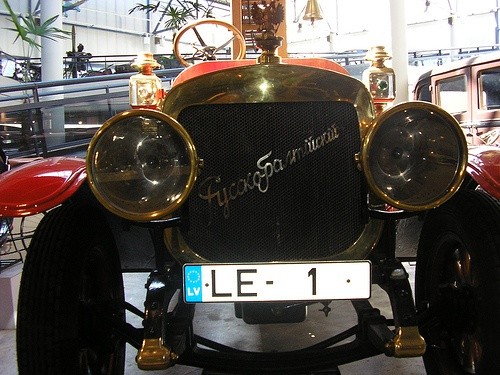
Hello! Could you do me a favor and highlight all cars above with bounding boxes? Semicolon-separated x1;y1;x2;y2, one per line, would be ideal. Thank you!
0;0;500;373
419;54;500;204
